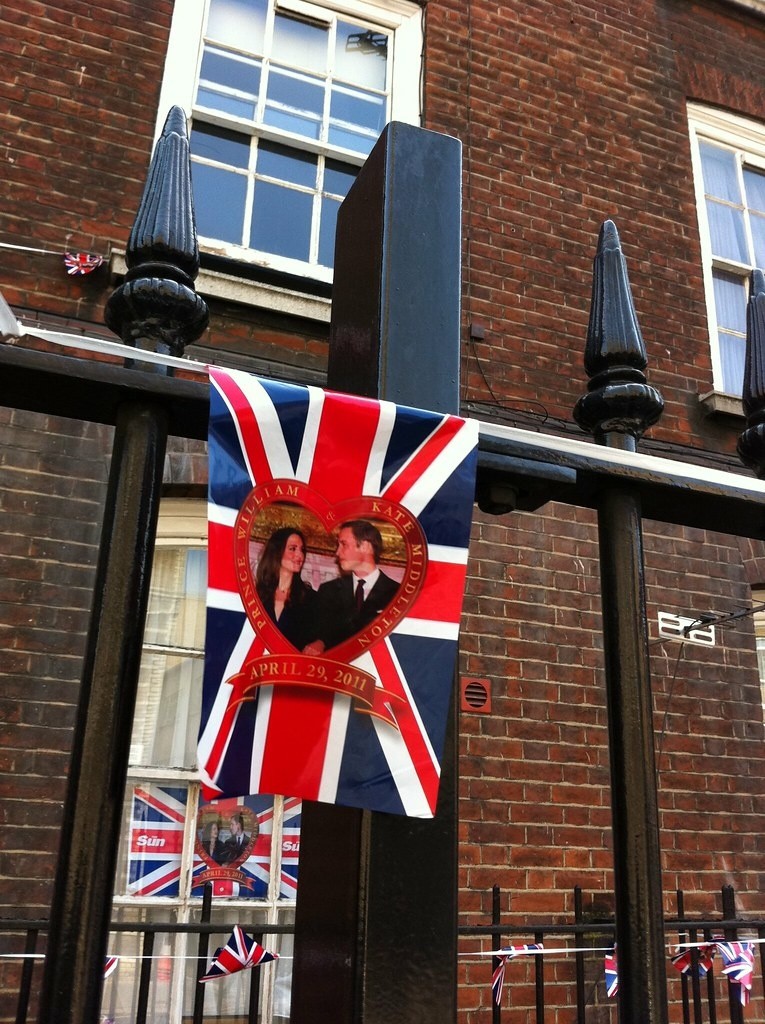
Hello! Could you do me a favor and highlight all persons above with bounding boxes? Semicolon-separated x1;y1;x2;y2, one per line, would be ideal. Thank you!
202;815;251;868
254;520;403;658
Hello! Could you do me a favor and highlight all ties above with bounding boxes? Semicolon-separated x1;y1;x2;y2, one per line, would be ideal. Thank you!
237;836;241;847
355;578;366;616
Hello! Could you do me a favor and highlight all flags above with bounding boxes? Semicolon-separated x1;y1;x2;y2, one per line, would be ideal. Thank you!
717;943;754;1005
491;943;543;1005
195;366;478;819
125;782;302;898
671;936;725;978
605;951;618;998
199;923;280;984
64;252;103;276
